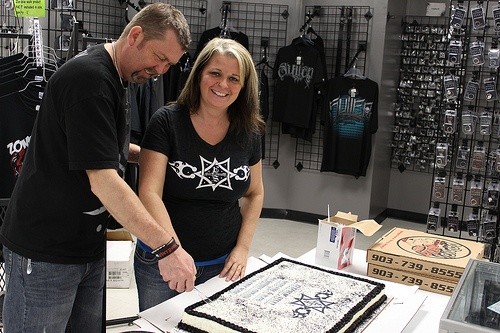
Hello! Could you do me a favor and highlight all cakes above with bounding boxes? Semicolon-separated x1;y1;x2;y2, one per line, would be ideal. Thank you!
176;257;387;333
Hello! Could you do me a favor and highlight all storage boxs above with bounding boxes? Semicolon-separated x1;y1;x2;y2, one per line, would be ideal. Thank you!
367;227;484;297
439;258;500;333
316;212;383;270
105;231;135;290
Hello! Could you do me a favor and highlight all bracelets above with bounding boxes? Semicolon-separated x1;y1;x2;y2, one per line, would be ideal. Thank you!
151;236;180;260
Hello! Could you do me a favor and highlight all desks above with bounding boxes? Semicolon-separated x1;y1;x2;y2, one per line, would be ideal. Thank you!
297;247;452;333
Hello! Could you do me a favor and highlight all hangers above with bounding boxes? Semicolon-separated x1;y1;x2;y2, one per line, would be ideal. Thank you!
344;57;365;79
254;56;273;71
20;23;61;92
219;20;238;39
292;18;317;45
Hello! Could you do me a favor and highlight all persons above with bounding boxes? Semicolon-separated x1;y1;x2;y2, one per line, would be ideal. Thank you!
132;37;265;312
0;1;197;331
